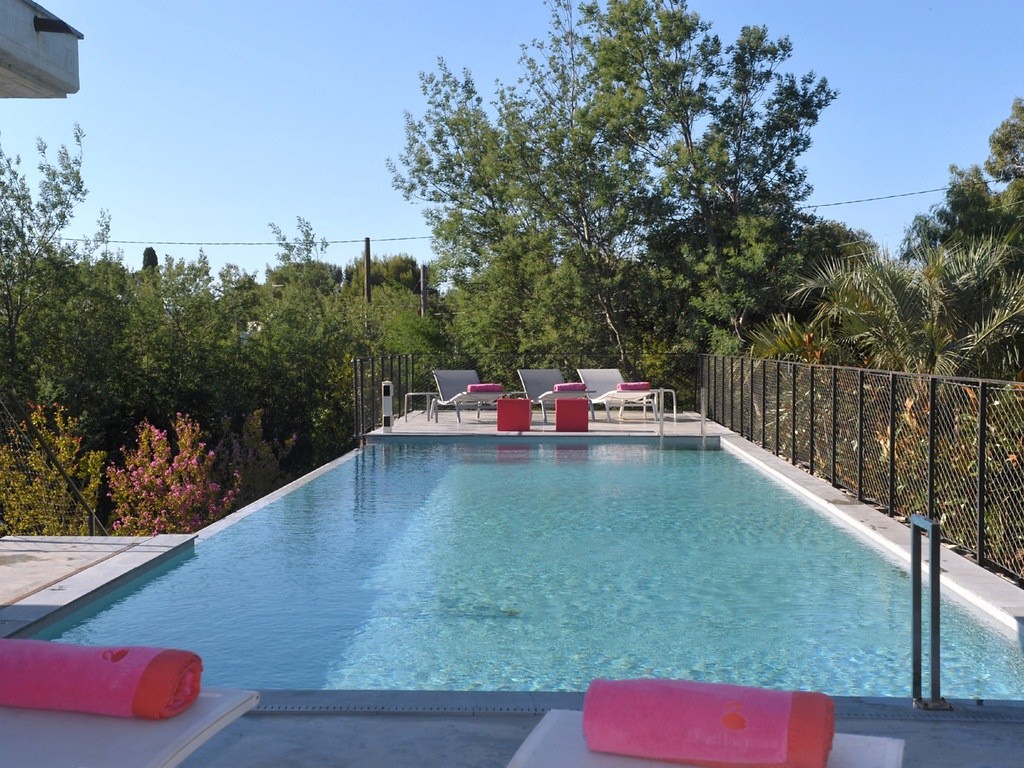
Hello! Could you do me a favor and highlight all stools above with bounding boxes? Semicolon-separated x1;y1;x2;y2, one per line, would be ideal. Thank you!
555;398;588;432
496;398;530;431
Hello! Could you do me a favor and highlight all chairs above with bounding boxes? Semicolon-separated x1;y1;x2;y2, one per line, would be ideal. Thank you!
424;368;530;424
0;685;261;768
499;706;907;768
517;369;597;425
575;369;678;423
